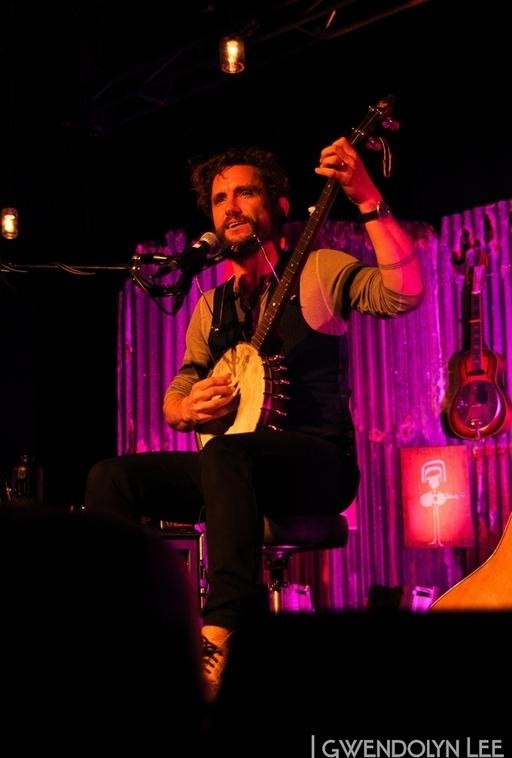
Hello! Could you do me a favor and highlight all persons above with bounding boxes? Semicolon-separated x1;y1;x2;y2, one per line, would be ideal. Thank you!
90;136;428;700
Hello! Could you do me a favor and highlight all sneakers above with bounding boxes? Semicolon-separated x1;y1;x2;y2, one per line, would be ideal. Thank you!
201;632;230;702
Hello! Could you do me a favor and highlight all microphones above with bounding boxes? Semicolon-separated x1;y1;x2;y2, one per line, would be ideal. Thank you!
150;228;219;280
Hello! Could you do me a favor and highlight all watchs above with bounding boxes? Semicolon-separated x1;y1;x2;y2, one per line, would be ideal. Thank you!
350;197;391;223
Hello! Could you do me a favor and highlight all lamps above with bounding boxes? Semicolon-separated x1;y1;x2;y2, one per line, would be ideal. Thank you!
218;35;247;76
1;207;19;242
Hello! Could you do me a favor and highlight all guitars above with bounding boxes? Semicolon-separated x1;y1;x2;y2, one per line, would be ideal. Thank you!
447;249;511;441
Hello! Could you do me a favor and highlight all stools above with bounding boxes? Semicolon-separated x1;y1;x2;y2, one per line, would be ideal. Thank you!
260;512;352;616
